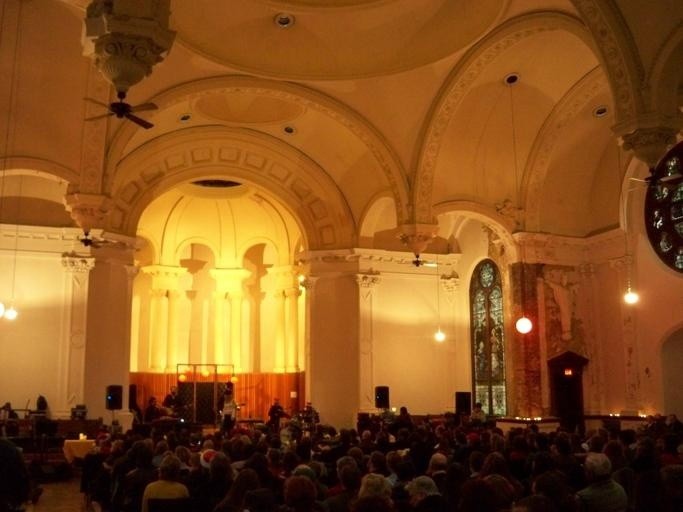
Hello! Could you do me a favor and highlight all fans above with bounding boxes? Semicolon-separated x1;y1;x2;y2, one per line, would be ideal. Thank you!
77;0;175;132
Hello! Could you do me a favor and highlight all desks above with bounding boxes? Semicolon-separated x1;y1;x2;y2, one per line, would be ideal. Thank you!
64;439;96;468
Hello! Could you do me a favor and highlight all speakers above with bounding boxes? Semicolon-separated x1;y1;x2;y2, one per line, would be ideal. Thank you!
106;385;122;409
375;386;389;408
